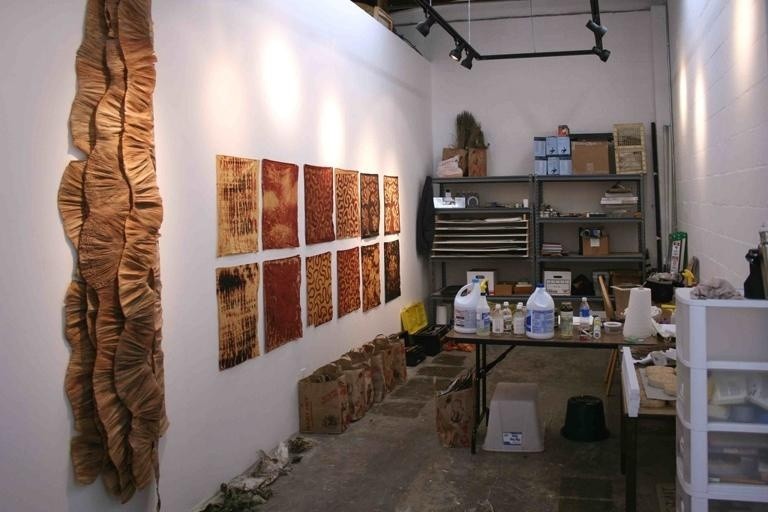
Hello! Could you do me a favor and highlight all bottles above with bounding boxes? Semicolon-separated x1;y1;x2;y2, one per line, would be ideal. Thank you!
477;292;490;336
560;302;573;338
503;302;512;331
492;304;504;336
594;317;601;339
513;305;525;335
555;308;558;327
518;302;526;314
579;297;589;329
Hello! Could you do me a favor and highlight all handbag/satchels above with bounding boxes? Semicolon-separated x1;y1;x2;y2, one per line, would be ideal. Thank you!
297;363;350;434
373;333;407;384
312;357;365;422
372;342;395;393
442;148;466;175
468;147;486;178
350;342;385;403
437;379;476;449
335;348;374;411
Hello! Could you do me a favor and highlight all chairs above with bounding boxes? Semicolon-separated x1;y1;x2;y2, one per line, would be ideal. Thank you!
597;275;624;396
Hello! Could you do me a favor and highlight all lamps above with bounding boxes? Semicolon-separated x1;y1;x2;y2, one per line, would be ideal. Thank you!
415;15;473;70
586;17;611;63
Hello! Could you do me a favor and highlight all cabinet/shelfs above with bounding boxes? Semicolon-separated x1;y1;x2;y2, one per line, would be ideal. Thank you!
674;287;768;512
428;173;534;324
535;176;646;311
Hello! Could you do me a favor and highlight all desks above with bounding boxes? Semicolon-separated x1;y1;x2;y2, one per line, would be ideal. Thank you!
445;317;676;453
619;365;677;512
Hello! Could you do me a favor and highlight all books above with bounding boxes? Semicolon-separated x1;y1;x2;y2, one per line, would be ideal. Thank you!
708;436;768;481
599;191;638;210
542;242;563;254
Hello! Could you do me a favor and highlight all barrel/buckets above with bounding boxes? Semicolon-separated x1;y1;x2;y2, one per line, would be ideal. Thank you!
453;279;481;333
525;284;555;340
560;396;610;442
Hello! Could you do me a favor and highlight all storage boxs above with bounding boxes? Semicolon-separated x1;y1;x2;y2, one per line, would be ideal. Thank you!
402;303;429;334
443;146;487;176
434;377;478;446
542;269;572;297
600;204;640;218
415;326;448;355
481;382;546;453
494;280;514;296
467;268;498;295
570;141;610;176
592;268;644;315
581;235;609;256
514;281;533;294
